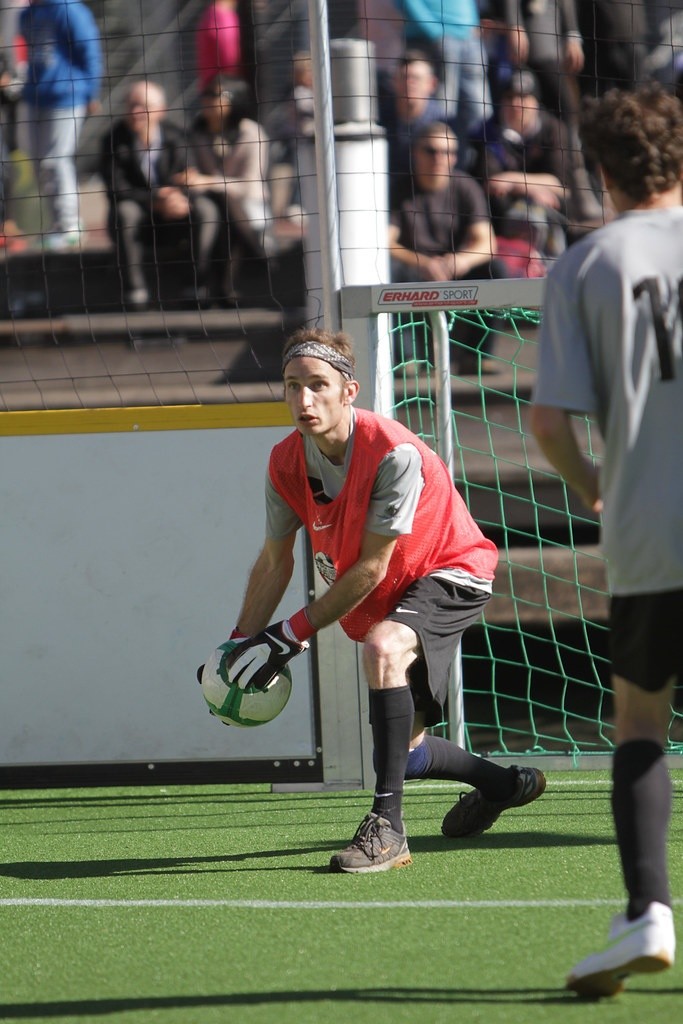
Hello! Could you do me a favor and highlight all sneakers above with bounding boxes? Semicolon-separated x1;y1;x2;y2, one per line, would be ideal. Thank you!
442;765;545;840
329;808;414;873
566;901;675;997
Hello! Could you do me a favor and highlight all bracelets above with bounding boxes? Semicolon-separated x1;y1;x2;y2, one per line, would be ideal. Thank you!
230;629;248;639
289;608;319;643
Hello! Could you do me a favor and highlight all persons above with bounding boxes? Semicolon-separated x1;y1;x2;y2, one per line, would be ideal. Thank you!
0;0;683;377
197;327;546;874
531;85;683;993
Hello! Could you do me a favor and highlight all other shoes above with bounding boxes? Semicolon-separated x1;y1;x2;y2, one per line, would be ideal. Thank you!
396;358;420;378
457;359;498;374
34;226;84;253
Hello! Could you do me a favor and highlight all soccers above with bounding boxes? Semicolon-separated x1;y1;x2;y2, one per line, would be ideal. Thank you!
200;636;292;727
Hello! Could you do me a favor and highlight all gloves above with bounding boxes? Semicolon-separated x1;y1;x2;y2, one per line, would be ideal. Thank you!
197;628;248;686
226;607;319;692
